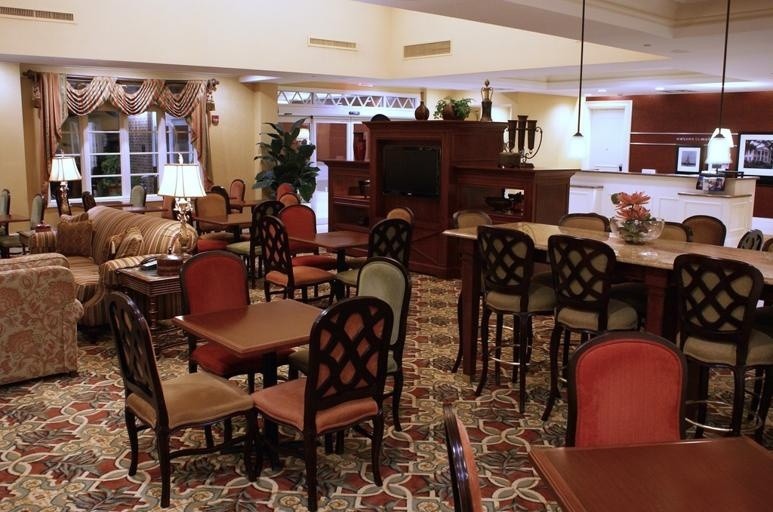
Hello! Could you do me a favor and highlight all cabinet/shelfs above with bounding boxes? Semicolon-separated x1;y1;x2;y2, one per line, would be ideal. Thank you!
319;158;370;257
449;161;581;277
355;116;509;278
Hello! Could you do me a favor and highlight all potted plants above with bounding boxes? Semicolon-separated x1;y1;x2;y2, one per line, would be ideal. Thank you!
434;93;476;118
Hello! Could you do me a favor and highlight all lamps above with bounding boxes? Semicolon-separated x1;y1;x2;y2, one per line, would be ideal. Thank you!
564;0;591;159
47;149;82;215
703;0;732;167
157;154;207;260
711;0;735;148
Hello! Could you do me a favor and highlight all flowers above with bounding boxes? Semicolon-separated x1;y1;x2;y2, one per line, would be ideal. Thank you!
615;193;651;223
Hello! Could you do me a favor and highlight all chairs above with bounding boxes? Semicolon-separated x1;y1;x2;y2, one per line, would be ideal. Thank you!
0;190;46;257
104;292;256;508
443;407;484;512
566;330;687;447
735;229;772;252
259;205;413;302
476;226;559;414
189;178;305;291
675;253;773;440
541;236;638;422
452;210;505;374
249;296;396;512
660;221;692;240
82;185;168;213
180;250;294;452
287;257;412;433
555;213;610;231
683;215;729;246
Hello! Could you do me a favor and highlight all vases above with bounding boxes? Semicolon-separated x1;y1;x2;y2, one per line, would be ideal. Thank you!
611;219;664;244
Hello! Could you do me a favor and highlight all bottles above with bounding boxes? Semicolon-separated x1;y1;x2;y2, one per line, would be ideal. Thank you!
352;131;368;160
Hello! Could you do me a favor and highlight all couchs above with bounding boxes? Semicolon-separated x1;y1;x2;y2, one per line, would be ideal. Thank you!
0;254;85;387
27;205;198;343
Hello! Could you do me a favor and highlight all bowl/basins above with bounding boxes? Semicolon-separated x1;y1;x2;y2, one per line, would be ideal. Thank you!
483;197;512;211
609;216;664;245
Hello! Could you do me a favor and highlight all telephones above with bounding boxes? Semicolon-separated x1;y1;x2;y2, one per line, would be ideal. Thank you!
140;256;157;271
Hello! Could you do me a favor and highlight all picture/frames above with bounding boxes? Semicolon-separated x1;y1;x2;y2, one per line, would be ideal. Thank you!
674;144;703;174
736;130;772;187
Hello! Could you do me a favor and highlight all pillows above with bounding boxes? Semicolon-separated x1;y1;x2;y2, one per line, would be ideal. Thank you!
99;234;122;261
115;224;145;259
54;221;94;258
60;212;88;222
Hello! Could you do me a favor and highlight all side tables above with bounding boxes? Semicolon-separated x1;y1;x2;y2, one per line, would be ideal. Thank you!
114;263;183;340
